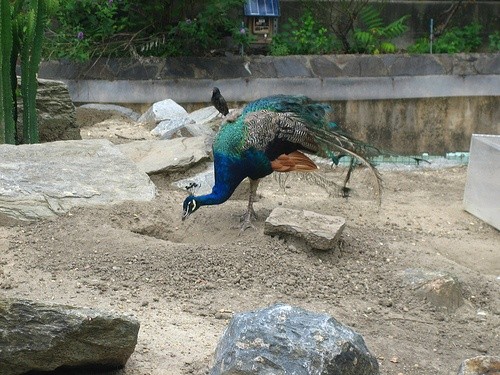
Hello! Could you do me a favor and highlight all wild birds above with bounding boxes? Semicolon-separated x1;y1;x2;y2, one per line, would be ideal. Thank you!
207;86;230;118
183;94;432;236
